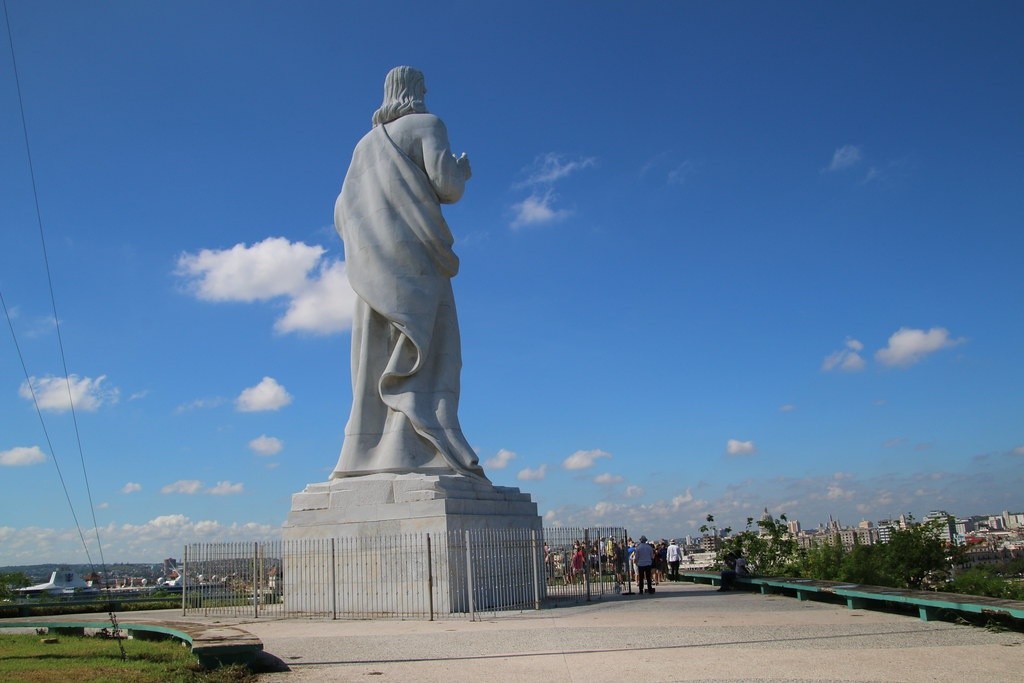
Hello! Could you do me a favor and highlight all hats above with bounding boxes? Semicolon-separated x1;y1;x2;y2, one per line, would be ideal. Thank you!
639;535;648;541
669;538;675;543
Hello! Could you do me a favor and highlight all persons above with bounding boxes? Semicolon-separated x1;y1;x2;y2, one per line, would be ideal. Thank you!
543;533;748;595
326;64;494;489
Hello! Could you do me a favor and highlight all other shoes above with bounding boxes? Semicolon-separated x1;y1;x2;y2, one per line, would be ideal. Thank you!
648;590;654;593
638;590;643;594
